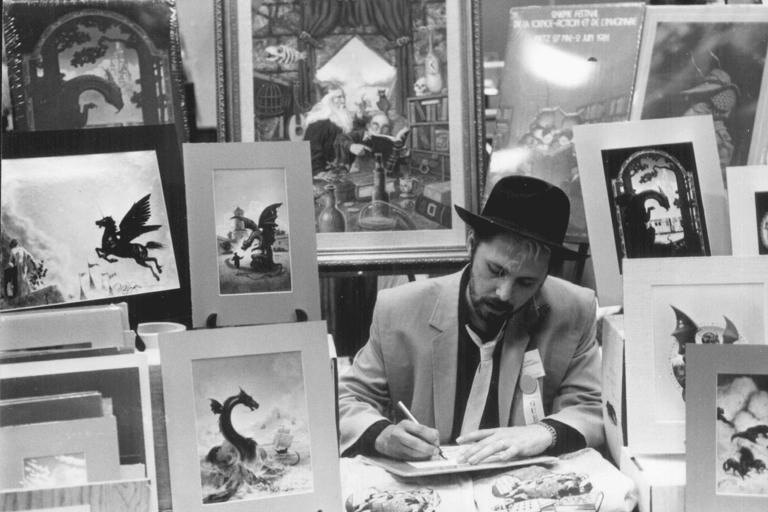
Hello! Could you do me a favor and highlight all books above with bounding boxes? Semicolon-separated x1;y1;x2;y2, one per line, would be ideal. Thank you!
0;303;160;512
366;125;410;175
404;98;448;153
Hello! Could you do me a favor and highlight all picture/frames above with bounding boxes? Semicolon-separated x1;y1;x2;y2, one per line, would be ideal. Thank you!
205;0;487;266
1;148;183;313
570;113;730;309
620;255;768;457
178;140;327;330
683;342;768;512
157;320;345;510
724;162;768;255
4;1;193;148
630;0;768;166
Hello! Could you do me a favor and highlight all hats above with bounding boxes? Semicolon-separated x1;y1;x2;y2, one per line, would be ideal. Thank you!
454;175;589;260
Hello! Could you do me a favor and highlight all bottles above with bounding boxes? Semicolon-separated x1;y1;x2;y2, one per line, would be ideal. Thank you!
423;25;445;94
369;151;390;218
317;183;347;233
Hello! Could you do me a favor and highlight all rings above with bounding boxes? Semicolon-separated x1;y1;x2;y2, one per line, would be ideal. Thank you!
498;439;506;451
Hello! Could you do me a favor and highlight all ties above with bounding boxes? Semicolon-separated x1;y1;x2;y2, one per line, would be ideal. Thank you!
460;320;510;443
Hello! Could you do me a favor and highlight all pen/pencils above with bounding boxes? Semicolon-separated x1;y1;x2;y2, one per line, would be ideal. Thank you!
396;401;445;459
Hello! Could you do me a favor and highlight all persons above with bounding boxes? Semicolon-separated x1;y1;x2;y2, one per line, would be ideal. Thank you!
301;86;364;177
336;174;602;468
331;113;395;176
1;237;41;300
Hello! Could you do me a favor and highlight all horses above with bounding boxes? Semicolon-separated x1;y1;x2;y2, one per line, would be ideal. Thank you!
96;217;168;280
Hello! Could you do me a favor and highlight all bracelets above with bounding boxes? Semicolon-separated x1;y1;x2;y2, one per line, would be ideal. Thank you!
530;419;557;452
